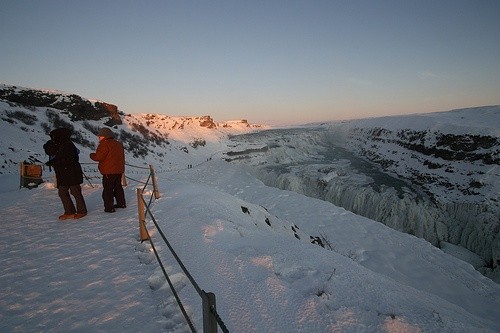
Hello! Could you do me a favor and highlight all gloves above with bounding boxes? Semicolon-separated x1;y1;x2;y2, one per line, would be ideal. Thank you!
45;160;54;166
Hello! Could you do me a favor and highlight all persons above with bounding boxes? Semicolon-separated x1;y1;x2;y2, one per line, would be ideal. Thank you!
42;127;90;222
88;127;127;214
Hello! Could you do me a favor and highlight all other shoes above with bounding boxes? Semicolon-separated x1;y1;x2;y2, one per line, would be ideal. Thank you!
59;213;76;220
104;209;115;212
114;205;127;208
74;213;87;219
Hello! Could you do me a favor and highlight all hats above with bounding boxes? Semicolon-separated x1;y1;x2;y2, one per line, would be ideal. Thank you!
97;128;114;136
